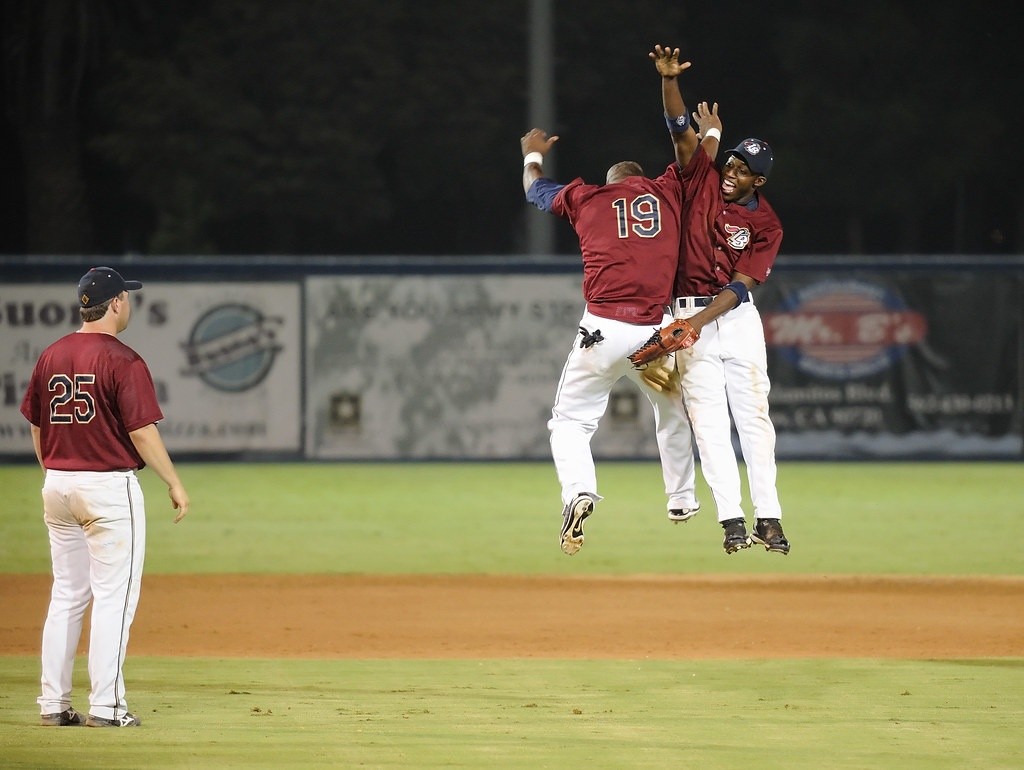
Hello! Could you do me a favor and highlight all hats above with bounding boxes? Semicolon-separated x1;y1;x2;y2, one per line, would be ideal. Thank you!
724;138;775;178
77;266;143;308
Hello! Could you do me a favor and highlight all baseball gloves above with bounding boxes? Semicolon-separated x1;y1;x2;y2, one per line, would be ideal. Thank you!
627;318;702;369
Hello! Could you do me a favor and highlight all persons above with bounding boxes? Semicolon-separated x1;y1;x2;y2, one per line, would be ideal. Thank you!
520;103;721;556
26;266;190;730
647;43;791;554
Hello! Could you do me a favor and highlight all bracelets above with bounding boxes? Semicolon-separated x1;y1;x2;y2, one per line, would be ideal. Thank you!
705;128;721;142
524;152;543;168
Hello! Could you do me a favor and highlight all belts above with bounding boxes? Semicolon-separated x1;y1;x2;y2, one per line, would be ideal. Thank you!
677;293;752;307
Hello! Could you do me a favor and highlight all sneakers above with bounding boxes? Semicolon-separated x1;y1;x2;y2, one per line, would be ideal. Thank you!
40;706;87;726
560;493;595;557
722;517;750;554
667;499;701;524
749;518;790;555
86;711;141;727
